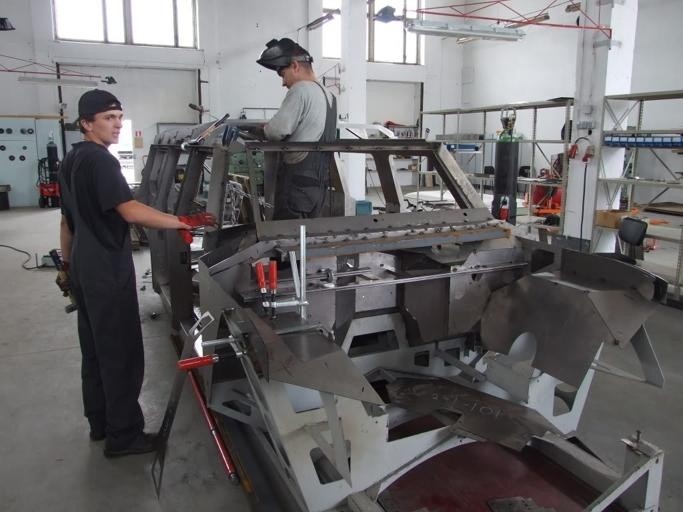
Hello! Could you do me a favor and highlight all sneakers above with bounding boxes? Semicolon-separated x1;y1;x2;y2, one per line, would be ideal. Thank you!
102;431;161;459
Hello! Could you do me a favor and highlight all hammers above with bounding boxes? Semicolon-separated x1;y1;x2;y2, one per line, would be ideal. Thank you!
49;249;79;313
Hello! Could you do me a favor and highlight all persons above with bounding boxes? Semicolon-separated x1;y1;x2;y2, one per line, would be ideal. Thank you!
257;44;345;219
54;90;214;456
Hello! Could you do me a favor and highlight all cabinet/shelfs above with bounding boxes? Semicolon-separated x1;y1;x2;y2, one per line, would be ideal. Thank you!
419;89;683;304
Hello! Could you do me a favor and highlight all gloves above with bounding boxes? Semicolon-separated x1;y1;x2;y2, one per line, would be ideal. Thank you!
177;211;217;229
55;261;73;297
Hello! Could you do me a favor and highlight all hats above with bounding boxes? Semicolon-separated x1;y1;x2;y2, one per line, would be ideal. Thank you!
76;88;123;123
